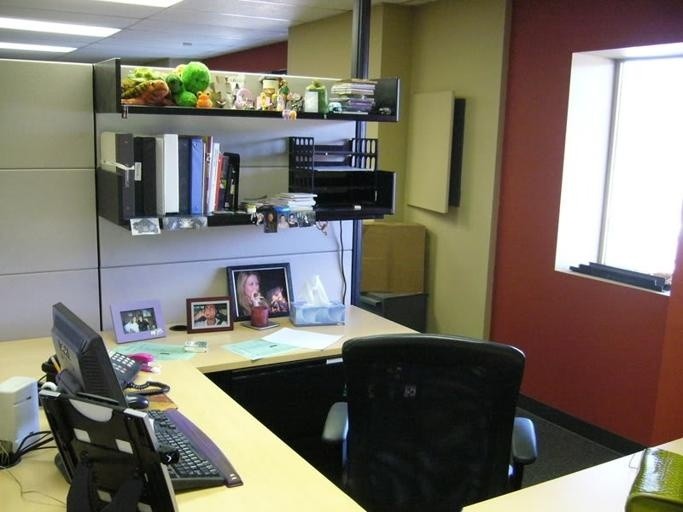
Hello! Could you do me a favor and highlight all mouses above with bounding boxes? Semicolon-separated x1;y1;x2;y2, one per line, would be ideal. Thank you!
123;393;149;409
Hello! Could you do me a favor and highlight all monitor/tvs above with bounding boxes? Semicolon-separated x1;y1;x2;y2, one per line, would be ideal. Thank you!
39;390;180;512
50;302;128;485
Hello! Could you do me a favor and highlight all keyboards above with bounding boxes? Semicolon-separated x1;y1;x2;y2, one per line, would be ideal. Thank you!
140;408;242;490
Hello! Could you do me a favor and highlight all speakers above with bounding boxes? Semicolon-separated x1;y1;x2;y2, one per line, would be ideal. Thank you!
0;376;40;452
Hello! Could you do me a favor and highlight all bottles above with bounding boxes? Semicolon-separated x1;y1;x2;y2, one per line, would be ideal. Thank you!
305;81;329;113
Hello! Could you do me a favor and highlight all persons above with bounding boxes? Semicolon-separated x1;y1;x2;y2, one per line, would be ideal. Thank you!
124;316;139;333
138;314;149;331
265;213;276;232
194;304;227;327
237;271;271;316
302;213;311;226
289;213;298;227
278;215;289;228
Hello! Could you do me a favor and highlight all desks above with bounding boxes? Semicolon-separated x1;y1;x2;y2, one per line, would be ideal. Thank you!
0;302;683;512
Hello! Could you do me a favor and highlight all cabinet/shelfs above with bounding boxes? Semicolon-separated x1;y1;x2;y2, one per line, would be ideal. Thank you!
92;56;399;232
355;292;428;331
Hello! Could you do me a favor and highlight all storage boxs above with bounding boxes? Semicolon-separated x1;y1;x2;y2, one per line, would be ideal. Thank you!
362;218;428;295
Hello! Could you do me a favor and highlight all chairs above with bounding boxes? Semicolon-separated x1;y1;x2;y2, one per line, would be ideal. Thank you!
321;333;538;512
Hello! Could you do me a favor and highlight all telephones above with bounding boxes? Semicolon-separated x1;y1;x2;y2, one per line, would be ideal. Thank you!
42;346;140;392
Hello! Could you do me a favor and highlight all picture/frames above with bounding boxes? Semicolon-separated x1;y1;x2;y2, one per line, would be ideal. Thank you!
109;298;166;344
226;262;294;322
185;297;234;332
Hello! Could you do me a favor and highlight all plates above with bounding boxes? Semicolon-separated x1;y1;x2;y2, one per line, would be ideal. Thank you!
242;319;279;330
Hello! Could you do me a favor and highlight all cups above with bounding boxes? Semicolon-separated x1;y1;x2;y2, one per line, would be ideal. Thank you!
262;80;279;103
302;91;319;113
250;306;269;326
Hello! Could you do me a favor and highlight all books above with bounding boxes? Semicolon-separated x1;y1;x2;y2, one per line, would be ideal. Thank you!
272;192;318;211
328;78;379;115
100;130;236;220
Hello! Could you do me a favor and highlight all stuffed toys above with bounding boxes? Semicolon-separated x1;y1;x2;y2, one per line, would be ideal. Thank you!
121;61;213;108
